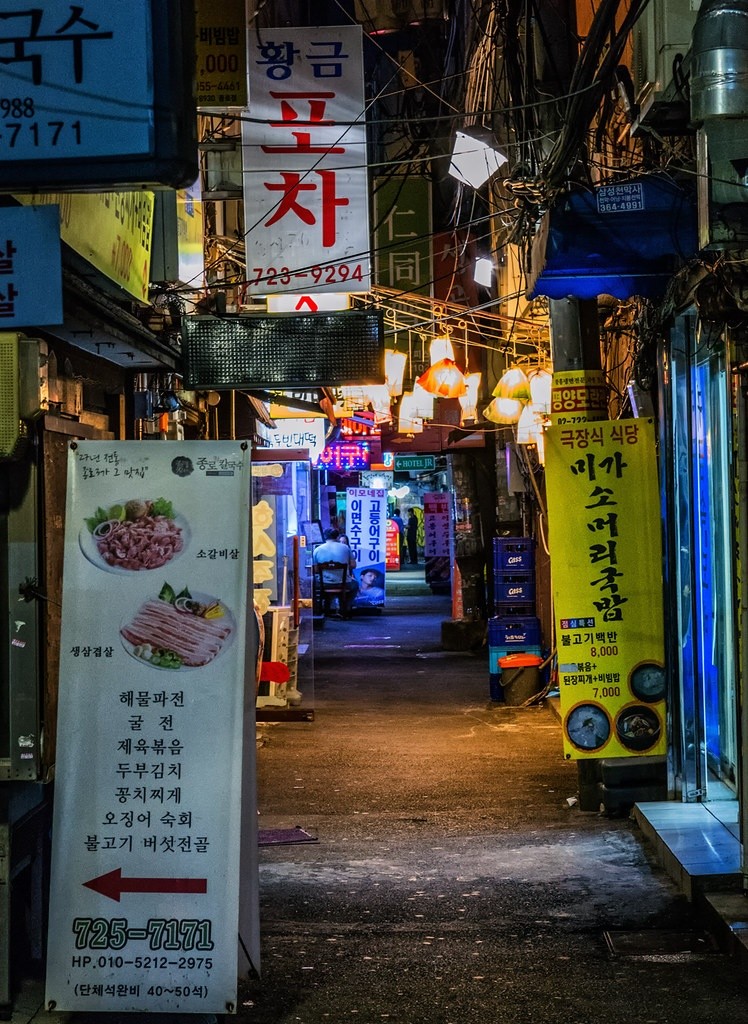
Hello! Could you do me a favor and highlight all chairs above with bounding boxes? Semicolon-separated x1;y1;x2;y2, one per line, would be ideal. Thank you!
313;559;348;617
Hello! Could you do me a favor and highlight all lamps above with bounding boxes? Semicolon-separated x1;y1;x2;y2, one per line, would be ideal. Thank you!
472;250;496;288
447;124;509;190
346;309;549;465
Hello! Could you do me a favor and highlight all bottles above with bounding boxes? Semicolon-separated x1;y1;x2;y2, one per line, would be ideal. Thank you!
493;608;531;629
505;544;527;552
504;576;528;583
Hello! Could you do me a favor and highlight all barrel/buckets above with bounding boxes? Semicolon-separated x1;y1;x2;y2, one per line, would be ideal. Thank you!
499;667;539;705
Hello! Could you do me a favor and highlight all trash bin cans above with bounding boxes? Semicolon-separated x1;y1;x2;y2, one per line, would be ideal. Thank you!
498;653;544;706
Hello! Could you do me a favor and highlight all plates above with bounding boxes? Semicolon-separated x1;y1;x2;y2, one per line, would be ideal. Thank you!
120;591;236;672
79;499;192;575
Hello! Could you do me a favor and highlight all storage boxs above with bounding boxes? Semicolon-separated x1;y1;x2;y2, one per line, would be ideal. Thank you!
486;535;541;698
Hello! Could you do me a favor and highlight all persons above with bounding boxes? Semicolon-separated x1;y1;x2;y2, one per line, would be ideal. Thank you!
355;568;384;605
312;527;360;622
405;508;418;564
390;509;405;565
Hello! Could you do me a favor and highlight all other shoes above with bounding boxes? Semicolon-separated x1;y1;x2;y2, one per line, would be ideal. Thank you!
407;561;417;564
